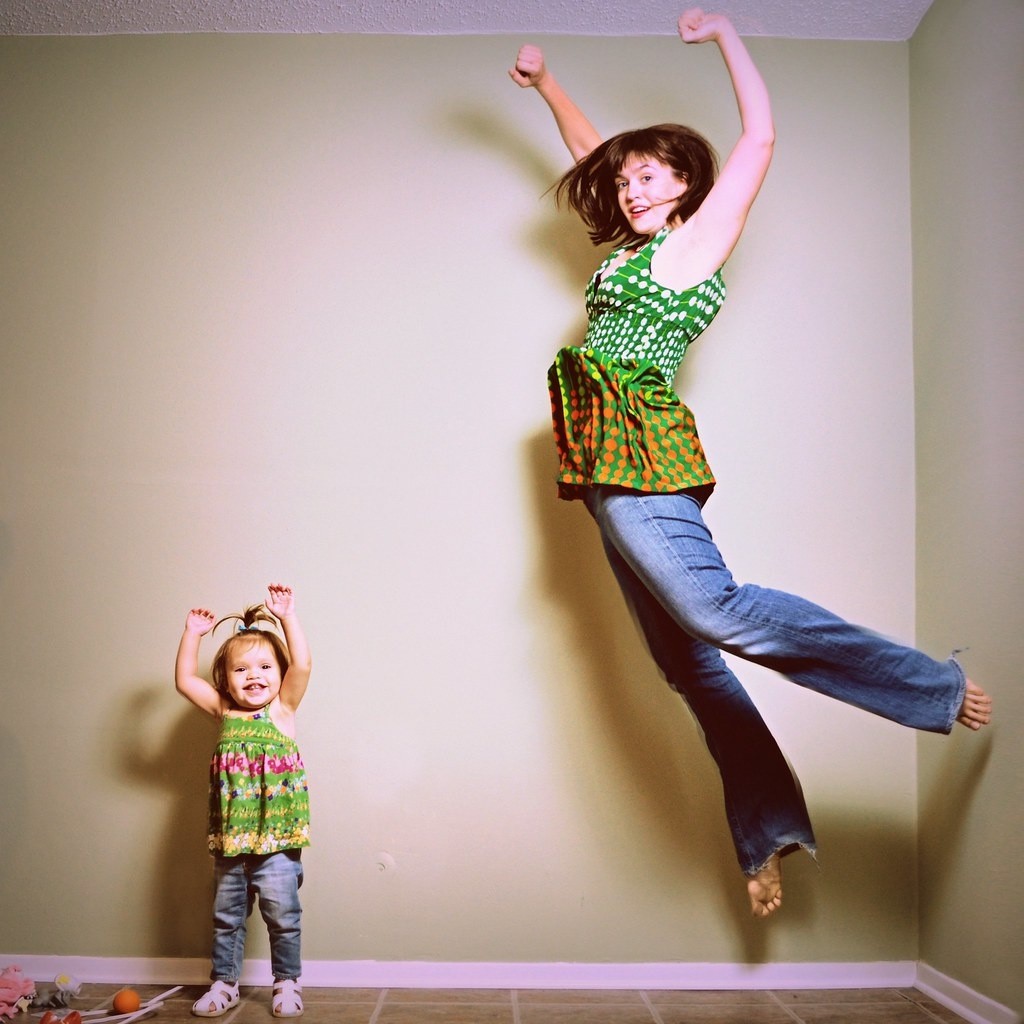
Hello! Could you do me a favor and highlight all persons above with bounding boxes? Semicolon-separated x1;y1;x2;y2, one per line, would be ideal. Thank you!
507;6;994;921
173;581;313;1018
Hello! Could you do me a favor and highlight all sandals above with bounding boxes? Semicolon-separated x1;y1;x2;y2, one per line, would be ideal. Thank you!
192;980;240;1017
272;979;304;1017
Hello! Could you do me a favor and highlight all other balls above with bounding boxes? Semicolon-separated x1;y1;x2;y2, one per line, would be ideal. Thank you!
113;990;140;1014
